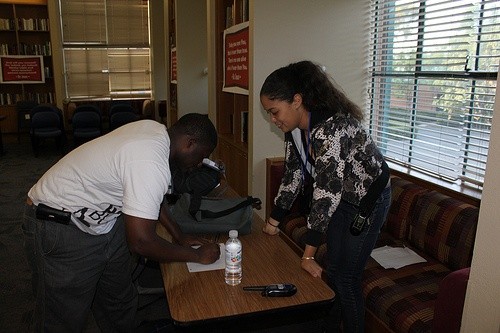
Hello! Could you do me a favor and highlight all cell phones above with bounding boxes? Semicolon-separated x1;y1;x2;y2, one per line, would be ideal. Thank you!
243;284;298;297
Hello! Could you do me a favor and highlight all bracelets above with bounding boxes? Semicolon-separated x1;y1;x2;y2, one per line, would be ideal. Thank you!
268;220;278;227
301;257;314;261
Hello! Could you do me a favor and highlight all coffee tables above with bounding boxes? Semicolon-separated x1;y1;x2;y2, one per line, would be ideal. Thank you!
154;179;336;329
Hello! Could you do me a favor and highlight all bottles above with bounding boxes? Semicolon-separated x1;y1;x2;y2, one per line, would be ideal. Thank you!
224;230;242;286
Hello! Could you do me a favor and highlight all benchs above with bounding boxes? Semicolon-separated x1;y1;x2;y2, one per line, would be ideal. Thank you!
265;157;481;333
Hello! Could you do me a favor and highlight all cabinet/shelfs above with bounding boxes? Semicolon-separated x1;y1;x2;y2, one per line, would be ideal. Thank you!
164;0;209;129
213;0;370;220
0;0;56;134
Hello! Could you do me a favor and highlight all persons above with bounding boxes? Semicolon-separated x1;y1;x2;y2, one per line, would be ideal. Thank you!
260;60;391;333
21;114;220;333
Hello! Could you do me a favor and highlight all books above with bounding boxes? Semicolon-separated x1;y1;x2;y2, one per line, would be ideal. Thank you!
0;18;56;105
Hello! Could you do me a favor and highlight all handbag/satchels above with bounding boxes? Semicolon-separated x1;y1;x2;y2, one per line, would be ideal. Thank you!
170;193;263;237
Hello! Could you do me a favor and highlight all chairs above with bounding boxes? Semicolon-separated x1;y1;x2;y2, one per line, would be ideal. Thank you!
27;103;139;159
